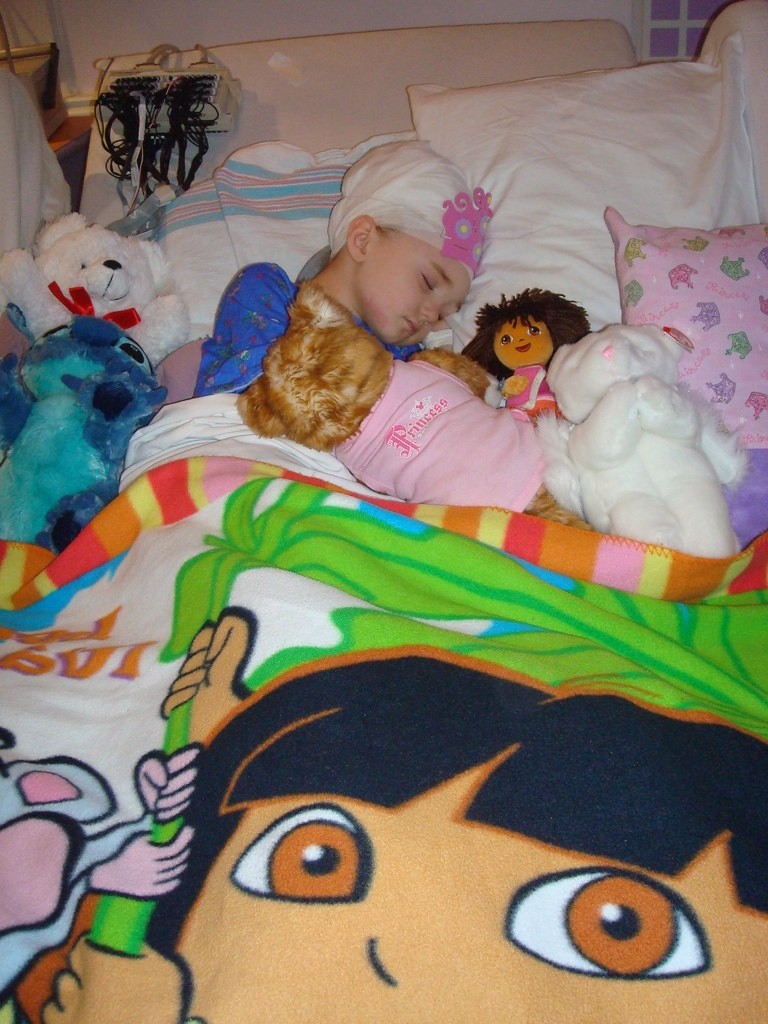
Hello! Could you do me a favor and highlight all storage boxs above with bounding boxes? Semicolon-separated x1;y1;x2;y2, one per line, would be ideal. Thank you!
0;42;71;142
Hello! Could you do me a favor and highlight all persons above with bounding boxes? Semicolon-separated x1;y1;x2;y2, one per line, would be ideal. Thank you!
192;142;481;400
461;287;593;428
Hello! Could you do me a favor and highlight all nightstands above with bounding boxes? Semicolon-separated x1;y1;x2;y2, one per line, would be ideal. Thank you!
47;116;100;214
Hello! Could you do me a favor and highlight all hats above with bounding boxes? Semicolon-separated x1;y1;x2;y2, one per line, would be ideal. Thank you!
328;141;493;291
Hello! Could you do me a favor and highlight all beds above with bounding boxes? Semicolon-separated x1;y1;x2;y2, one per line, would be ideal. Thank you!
0;0;768;1024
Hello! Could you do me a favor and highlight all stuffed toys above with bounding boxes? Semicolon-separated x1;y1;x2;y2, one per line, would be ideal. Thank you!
534;324;745;558
0;316;167;554
238;280;593;532
0;213;190;368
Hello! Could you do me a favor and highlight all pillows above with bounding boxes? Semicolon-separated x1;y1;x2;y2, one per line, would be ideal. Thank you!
403;29;761;361
603;203;768;448
